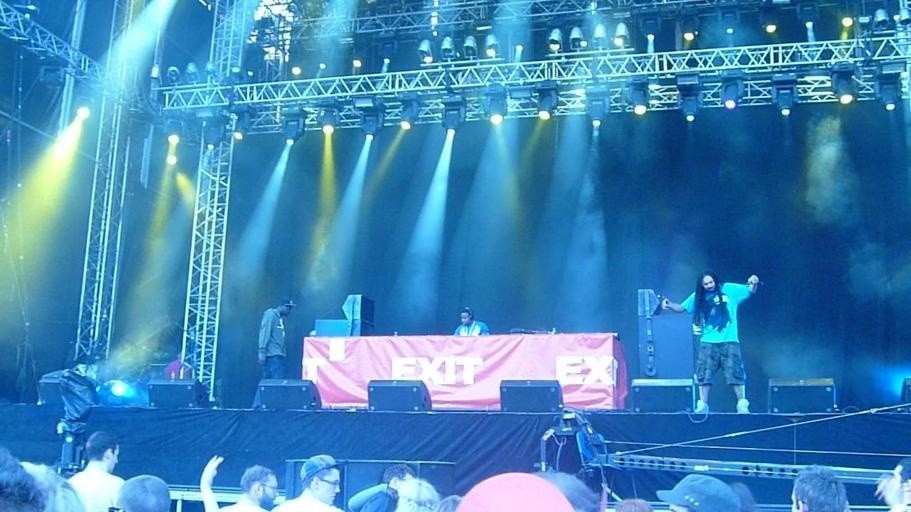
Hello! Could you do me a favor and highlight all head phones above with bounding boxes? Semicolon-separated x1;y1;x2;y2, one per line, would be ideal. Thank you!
464;306;474;320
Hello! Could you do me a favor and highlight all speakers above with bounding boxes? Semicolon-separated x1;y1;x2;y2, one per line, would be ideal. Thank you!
147;378;209;408
285;459;344;511
258;378;322;409
38;370;64;405
631;378;695;413
500;379;562;412
344;461;417;512
413;461;456;498
367;379;432;411
768;378;835;413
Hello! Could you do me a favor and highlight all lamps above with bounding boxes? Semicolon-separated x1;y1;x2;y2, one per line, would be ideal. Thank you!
867;5;911;29
482;82;514;126
201;116;232;150
157;106;190;147
579;84;615;128
543;19;636;56
348;90;390;146
229;104;257;142
672;69;707;124
151;61;221;87
532;76;560;124
826;61;861;108
871;56;909;113
311;97;344;136
277;107;310;146
721;66;753;112
414;30;502;63
620;74;656;116
767;68;806;118
438;92;472;135
395;88;426;133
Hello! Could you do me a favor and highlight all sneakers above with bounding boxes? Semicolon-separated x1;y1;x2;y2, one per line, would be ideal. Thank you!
736;399;749;414
694;400;709;413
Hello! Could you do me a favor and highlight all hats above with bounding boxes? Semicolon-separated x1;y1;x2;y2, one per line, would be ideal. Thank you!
279;297;294;306
655;474;741;512
299;455;348;484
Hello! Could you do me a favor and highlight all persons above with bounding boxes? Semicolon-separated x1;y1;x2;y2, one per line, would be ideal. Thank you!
0;430;911;512
661;270;759;415
453;307;490;336
162;351;191;380
256;296;296;409
